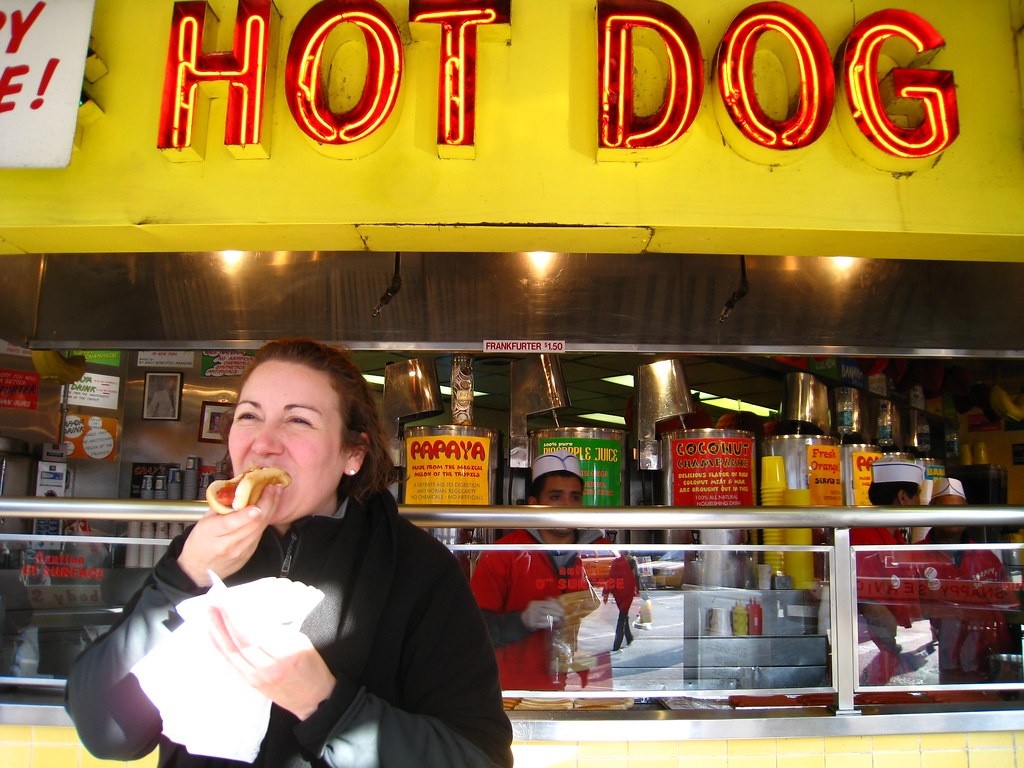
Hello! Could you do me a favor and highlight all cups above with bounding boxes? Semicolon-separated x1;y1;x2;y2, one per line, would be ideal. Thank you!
709;608;731;635
760;456;813;590
125;521;194;567
140;456;213;500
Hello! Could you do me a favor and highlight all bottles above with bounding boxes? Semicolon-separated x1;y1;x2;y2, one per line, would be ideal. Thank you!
748;597;763;635
732;600;748;635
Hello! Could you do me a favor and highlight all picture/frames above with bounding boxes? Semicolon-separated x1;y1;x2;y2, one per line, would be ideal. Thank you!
142;371;183;421
198;401;237;445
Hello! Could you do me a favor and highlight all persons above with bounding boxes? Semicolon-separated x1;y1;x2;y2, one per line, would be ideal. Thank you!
63;338;514;768
211;415;220;429
469;450;633;692
849;459;1024;685
602;549;649;651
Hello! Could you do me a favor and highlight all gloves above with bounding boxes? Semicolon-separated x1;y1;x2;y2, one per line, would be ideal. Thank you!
577;620;627;657
521;601;564;630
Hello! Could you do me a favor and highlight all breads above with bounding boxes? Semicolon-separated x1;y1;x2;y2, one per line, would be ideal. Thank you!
500;697;635;710
549;589;603;672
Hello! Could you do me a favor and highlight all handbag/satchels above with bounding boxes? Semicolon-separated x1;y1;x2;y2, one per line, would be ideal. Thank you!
632;600;652;632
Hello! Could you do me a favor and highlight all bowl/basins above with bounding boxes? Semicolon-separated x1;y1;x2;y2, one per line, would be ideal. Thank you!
987;654;1024;700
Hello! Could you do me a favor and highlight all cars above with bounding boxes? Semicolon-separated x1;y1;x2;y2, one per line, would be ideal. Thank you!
635;552;686;590
577;548;621;585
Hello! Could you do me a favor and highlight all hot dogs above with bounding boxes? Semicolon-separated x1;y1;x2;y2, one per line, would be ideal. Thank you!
206;466;290;515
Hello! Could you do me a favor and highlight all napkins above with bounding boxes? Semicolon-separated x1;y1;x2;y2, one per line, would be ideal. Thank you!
131;569;324;762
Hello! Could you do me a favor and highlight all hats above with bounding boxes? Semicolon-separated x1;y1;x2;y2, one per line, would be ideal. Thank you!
532;450;583;482
931;478;966;499
871;458;926;486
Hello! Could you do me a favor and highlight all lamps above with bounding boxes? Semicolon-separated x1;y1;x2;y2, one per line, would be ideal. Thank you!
371;252;402;318
719;255;748;324
